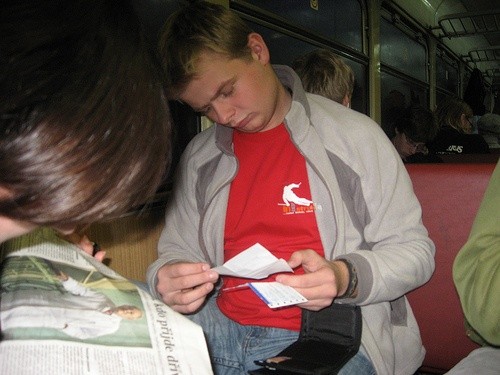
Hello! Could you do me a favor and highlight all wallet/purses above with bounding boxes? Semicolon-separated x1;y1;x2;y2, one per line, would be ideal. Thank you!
246;299;362;375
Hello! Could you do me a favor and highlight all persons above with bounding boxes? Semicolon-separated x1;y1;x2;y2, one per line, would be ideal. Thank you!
0;270;142;341
285;49;500;375
0;0;171;375
145;0;437;375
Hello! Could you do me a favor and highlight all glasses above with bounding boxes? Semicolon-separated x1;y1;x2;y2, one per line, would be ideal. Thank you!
405;134;424;151
466;117;475;130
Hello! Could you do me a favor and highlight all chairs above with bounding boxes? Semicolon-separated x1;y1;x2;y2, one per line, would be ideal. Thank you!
398;162;497;370
